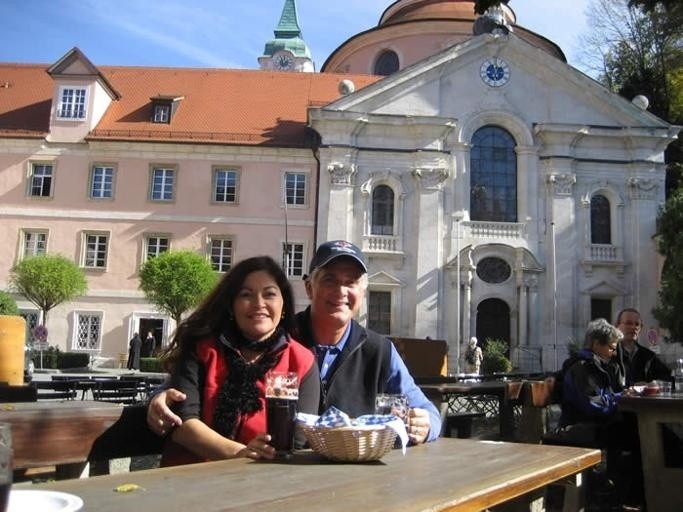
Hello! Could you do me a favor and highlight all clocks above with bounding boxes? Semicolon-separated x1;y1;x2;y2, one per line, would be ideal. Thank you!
480;58;510;88
275;54;292;70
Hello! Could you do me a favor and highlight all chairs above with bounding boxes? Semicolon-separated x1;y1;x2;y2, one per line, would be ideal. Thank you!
409;365;683;511
0;366;174;405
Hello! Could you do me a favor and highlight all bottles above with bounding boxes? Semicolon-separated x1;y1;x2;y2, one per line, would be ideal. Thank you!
675;358;683;399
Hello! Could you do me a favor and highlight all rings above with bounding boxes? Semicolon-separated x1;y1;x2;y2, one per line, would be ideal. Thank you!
413;426;421;434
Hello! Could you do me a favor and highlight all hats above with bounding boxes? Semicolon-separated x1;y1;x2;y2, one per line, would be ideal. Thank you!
309;240;368;274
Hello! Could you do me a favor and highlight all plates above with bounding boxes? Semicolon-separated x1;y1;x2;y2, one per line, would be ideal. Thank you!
5;489;84;512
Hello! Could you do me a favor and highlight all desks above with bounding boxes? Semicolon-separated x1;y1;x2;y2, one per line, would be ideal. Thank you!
0;399;605;512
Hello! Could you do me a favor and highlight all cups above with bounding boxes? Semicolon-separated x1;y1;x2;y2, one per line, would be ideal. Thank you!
374;393;410;450
0;421;14;512
264;371;299;461
658;381;672;397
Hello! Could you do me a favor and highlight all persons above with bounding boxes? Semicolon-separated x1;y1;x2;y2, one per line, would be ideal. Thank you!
141;329;156;358
24;344;35;384
143;238;444;454
656;321;664;338
550;316;657;512
126;331;143;371
463;335;483;376
542;306;682;485
646;325;658;346
158;254;321;471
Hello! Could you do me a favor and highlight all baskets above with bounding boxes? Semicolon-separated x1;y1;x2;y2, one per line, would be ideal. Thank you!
301;426;397;462
